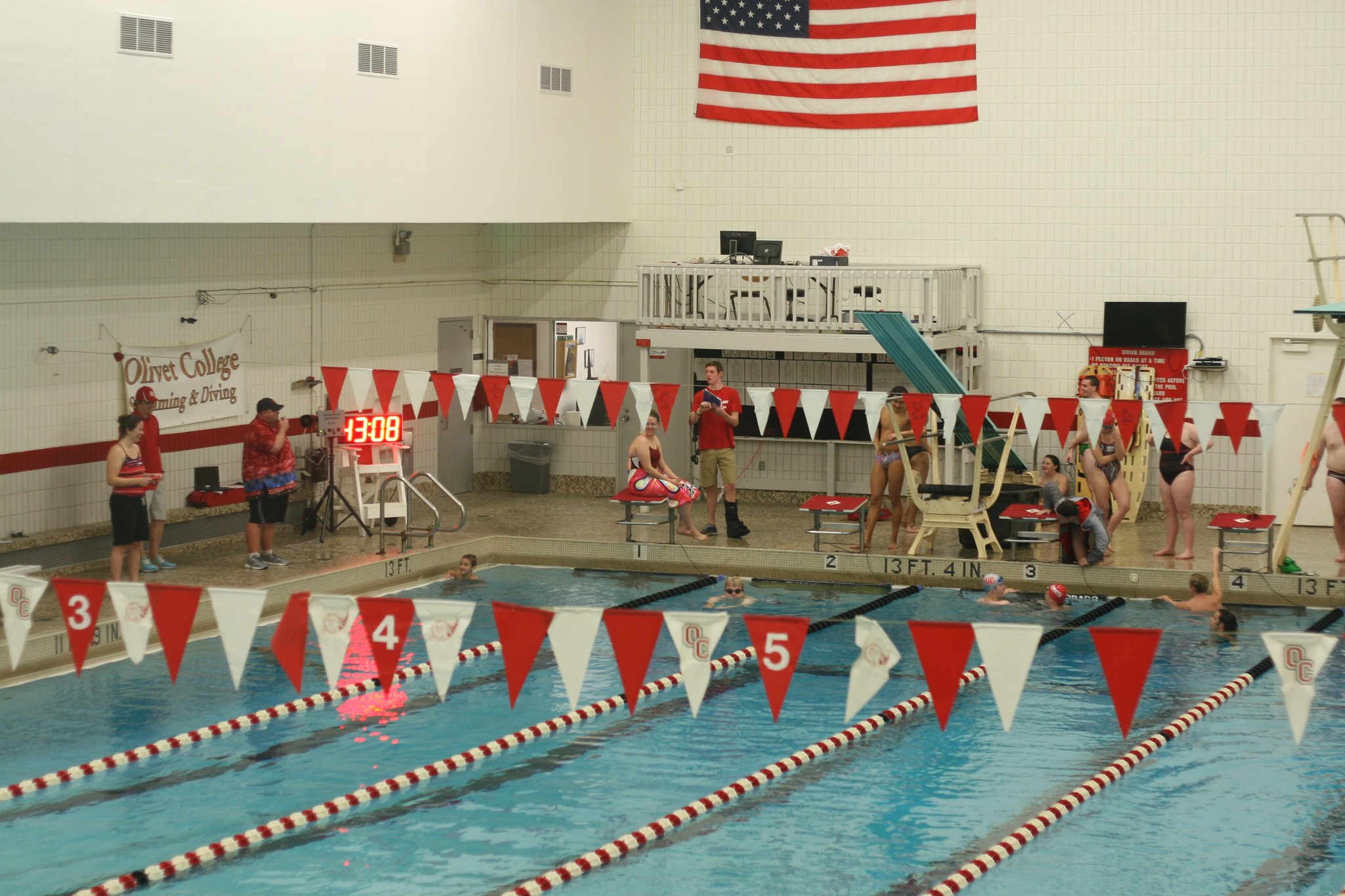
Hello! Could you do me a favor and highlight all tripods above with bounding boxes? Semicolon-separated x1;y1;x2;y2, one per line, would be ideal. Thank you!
298;437;373;544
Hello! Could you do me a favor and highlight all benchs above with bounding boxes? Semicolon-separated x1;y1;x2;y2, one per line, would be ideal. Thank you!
1;489;312;590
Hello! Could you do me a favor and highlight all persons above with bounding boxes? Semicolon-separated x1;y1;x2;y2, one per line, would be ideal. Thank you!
975;573;1020;607
1087;409;1132;557
1153;547;1223;613
1145;422;1214;561
1038;454;1071;504
1043;583;1068;609
117;384;178;575
1064;375;1104;506
106;414;164;584
848;385;927;551
443;554;481;582
902;437;934;533
688;360;752;540
1036;481;1112;568
1209;609;1238;638
1300;396;1345;565
241;398;299;572
627;409;710;542
705;573;759;611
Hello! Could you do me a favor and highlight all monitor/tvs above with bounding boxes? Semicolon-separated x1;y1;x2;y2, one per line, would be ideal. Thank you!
752;239;783;265
1104;301;1187;349
719;230;757;257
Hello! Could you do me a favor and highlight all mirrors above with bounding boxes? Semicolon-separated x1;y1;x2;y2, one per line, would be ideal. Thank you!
563;340;578;379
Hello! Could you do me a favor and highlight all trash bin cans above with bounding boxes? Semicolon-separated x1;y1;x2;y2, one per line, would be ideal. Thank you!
507;441;553;495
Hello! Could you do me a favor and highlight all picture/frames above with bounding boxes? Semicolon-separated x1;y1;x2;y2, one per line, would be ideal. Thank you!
584;350;595;367
576;327;586;344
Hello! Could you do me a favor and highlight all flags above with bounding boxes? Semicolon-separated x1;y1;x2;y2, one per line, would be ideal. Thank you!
694;1;980;133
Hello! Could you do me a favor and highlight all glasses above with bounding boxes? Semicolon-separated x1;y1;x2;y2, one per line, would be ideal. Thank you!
724;588;745;594
646;423;658;427
1065;461;1076;475
1102;425;1114;429
997;576;1003;583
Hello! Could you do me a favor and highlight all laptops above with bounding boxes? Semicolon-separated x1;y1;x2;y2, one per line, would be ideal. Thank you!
192;465;234;492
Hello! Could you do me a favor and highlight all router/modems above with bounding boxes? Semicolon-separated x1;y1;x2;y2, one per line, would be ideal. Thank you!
1188;357;1228;370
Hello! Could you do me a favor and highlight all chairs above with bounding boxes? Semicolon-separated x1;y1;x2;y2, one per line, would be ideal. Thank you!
723;276;771;321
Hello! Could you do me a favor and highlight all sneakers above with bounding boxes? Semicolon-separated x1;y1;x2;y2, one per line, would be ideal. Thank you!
138;557;160;572
148;554;177;569
245;554;269;570
699;523;718;536
260;553;289;565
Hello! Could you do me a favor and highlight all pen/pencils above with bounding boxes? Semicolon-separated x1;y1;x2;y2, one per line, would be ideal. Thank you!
698;404;704;407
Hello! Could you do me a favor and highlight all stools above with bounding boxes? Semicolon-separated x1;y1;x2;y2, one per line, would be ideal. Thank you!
850;286;882;310
785;289;814;322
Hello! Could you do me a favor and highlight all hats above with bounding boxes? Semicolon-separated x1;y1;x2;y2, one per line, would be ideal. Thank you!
256;397;284;414
1103;408;1115;425
982;573;1004;592
1049;583;1068;605
135;386;160;404
